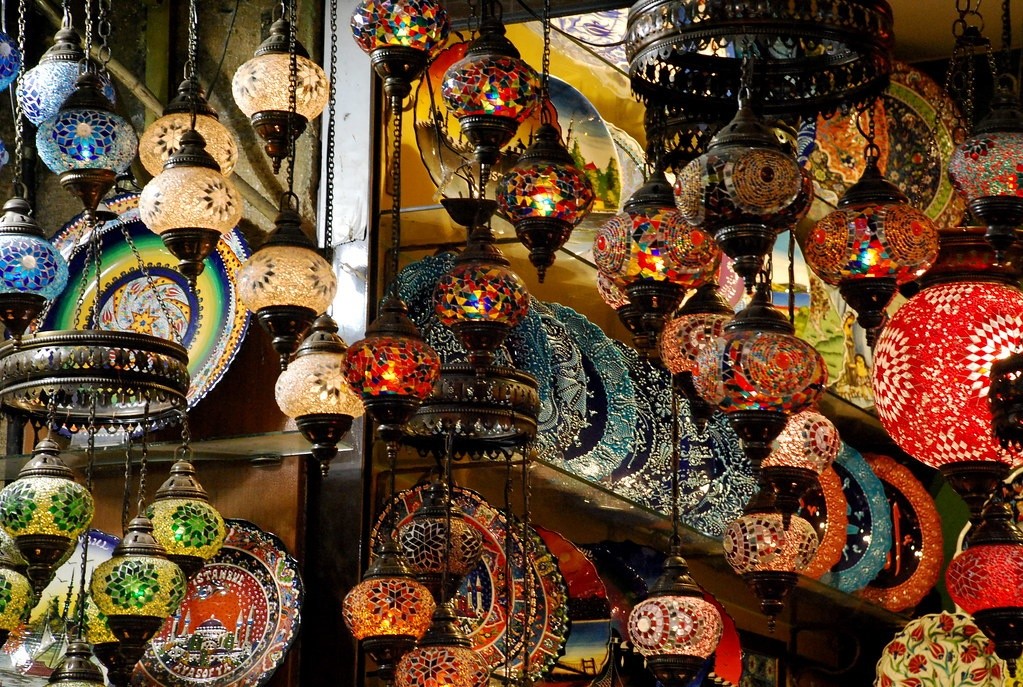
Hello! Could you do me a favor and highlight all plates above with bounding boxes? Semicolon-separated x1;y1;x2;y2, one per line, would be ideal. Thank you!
365;251;946;687
128;518;304;687
5;192;253;439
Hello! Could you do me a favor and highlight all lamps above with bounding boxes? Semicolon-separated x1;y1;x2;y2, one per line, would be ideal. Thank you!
0;1;1023;687
